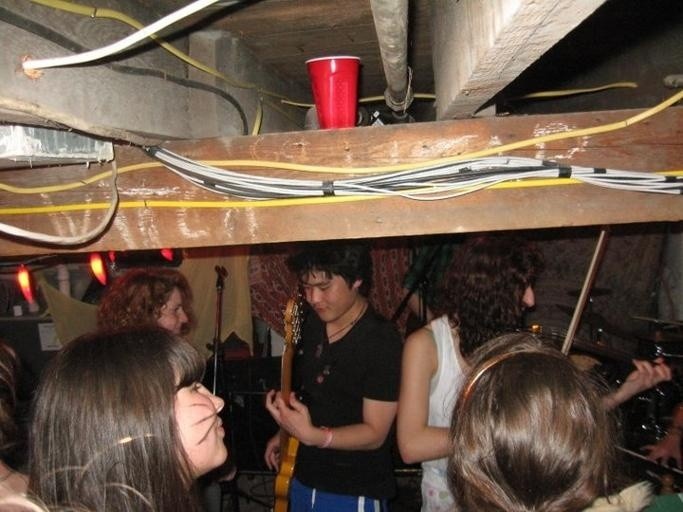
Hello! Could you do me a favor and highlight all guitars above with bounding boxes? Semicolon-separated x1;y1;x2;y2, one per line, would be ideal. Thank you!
273;300;304;512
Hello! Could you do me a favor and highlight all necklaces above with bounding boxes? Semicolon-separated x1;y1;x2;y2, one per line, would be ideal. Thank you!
328;300;366;340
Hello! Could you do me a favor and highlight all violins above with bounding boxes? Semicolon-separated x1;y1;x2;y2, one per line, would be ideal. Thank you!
508;322;681;389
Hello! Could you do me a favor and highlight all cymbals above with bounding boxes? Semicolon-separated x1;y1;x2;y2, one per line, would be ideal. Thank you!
555;303;636;348
631;315;683;325
567;288;611;297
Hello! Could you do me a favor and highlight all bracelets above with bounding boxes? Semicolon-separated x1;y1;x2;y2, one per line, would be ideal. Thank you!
317;426;333;450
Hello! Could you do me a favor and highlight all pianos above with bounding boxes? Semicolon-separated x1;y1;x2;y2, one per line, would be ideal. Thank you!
601;430;683;490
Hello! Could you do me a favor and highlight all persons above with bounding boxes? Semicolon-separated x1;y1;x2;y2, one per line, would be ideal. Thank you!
0;339;46;511
639;401;683;471
26;326;229;512
94;266;237;512
264;238;403;512
397;234;672;512
446;334;683;511
